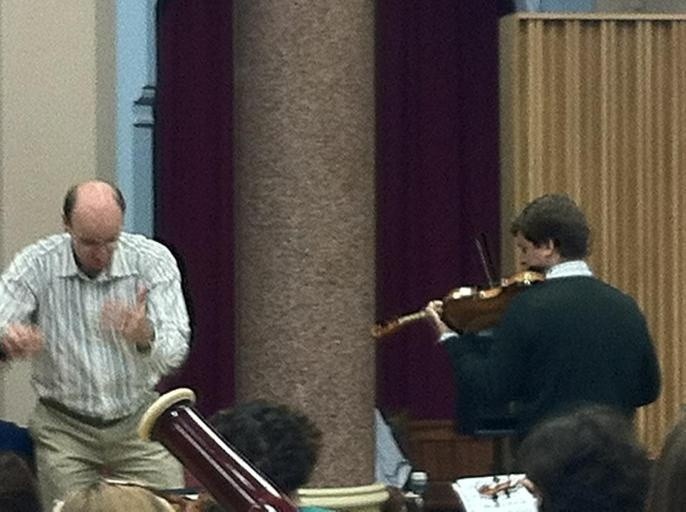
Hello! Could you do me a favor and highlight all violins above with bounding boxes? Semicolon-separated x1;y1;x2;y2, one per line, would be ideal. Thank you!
368;267;545;341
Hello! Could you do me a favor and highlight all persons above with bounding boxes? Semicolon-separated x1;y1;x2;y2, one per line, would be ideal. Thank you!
652;415;686;509
2;400;337;512
0;177;191;512
424;190;664;470
517;400;651;512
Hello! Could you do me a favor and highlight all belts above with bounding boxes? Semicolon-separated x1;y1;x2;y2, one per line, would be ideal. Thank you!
37;396;128;429
